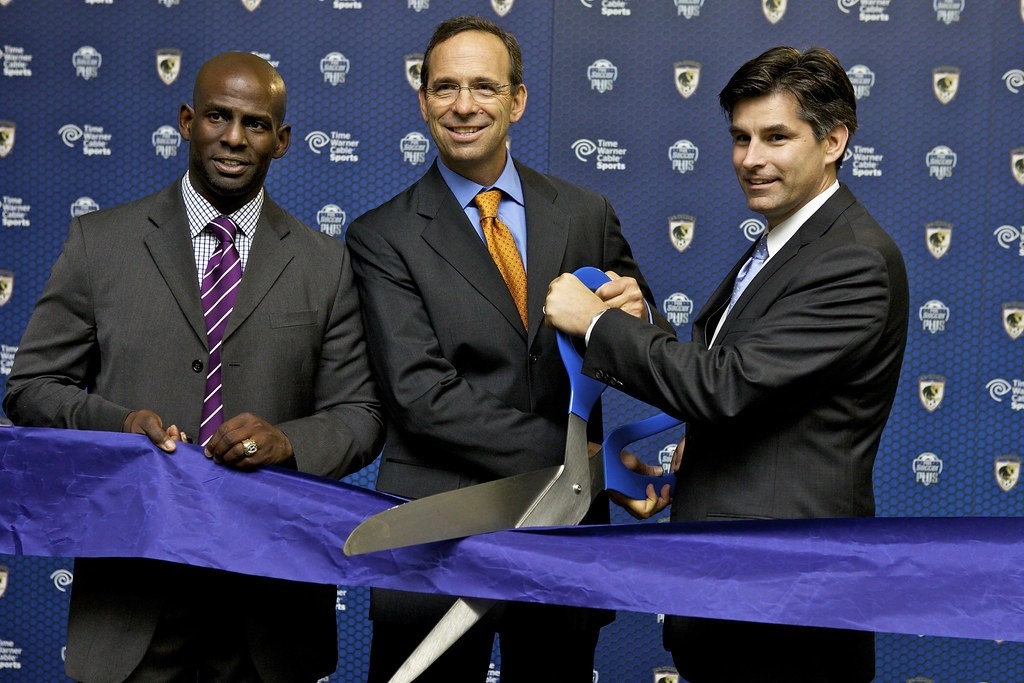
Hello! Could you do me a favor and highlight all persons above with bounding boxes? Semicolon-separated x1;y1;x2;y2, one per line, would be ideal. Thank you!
542;41;911;683
344;13;675;683
1;49;390;683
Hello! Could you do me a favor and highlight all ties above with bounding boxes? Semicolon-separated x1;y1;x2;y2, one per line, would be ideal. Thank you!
726;235;769;314
473;189;527;330
195;214;242;445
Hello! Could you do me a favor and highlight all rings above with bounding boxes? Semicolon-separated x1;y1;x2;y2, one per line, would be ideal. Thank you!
241;438;258;456
542;305;546;315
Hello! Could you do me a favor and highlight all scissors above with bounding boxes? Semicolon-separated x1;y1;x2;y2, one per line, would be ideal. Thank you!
342;266;697;683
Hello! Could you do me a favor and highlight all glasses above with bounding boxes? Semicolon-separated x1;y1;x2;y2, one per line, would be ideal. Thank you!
423;80;515;104
